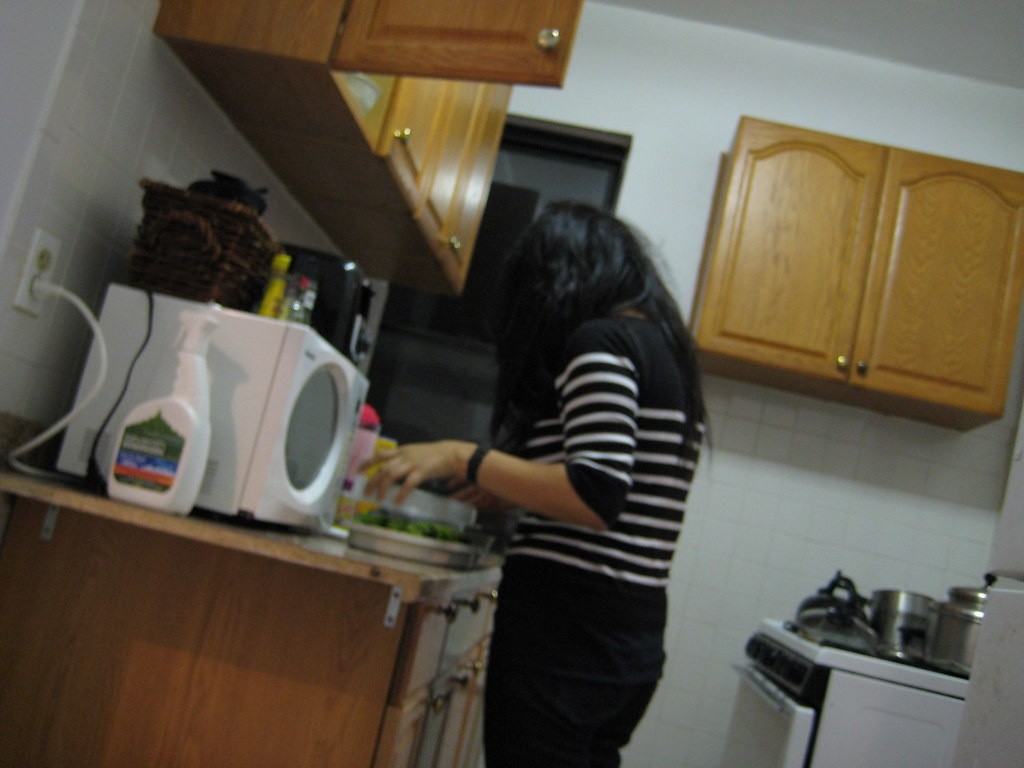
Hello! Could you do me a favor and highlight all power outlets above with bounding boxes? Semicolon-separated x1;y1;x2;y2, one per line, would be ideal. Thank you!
11;228;63;316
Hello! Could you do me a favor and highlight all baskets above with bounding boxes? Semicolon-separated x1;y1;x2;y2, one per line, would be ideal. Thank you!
121;177;284;311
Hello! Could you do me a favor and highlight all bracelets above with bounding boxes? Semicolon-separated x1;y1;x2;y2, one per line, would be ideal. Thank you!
466;441;492;485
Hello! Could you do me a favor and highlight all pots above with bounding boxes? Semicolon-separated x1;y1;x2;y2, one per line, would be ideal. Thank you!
930;570;998;675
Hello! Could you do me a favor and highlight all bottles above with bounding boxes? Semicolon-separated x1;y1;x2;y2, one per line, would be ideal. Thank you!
256;253;310;323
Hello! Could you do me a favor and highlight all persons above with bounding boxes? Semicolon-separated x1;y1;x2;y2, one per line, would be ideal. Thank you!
354;199;707;768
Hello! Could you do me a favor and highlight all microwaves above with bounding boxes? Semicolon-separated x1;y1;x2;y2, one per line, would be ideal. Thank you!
54;285;370;531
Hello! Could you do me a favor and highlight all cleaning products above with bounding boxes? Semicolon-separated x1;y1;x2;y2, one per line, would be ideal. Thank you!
107;310;219;516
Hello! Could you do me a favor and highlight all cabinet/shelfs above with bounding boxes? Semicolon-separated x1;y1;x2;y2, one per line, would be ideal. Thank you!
151;0;586;300
688;116;1023;433
1;471;507;768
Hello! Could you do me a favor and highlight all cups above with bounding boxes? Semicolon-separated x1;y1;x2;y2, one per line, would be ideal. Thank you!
871;589;941;665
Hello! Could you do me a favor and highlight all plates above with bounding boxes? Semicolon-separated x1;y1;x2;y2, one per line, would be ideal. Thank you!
345;522;478;569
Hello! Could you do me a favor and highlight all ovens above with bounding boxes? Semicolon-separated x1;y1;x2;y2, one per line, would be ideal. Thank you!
719;622;970;768
